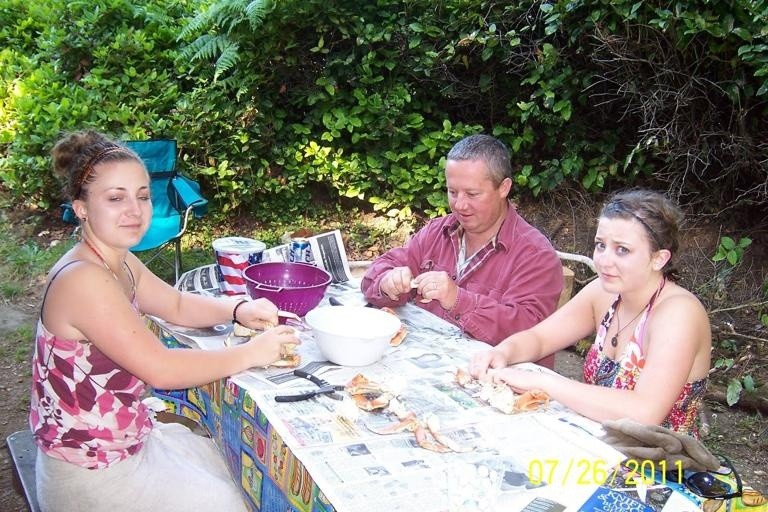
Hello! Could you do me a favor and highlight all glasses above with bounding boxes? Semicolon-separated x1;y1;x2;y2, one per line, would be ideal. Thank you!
666;453;743;500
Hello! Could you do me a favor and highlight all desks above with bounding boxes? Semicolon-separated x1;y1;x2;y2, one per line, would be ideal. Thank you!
166;274;768;512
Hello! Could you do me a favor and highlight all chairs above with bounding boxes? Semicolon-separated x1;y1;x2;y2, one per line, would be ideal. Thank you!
55;139;208;282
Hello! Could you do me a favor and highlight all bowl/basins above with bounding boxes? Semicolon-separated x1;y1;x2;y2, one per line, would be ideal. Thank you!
304;305;402;366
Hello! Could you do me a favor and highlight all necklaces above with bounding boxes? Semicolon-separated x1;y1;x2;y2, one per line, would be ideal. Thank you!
610;304;646;347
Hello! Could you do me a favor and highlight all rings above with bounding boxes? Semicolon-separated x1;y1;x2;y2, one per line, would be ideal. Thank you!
433;282;437;289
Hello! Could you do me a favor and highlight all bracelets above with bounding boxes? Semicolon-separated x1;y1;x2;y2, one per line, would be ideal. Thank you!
231;300;248;325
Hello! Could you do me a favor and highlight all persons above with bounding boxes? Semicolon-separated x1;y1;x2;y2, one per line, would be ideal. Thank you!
360;133;565;373
468;190;712;441
27;129;301;512
